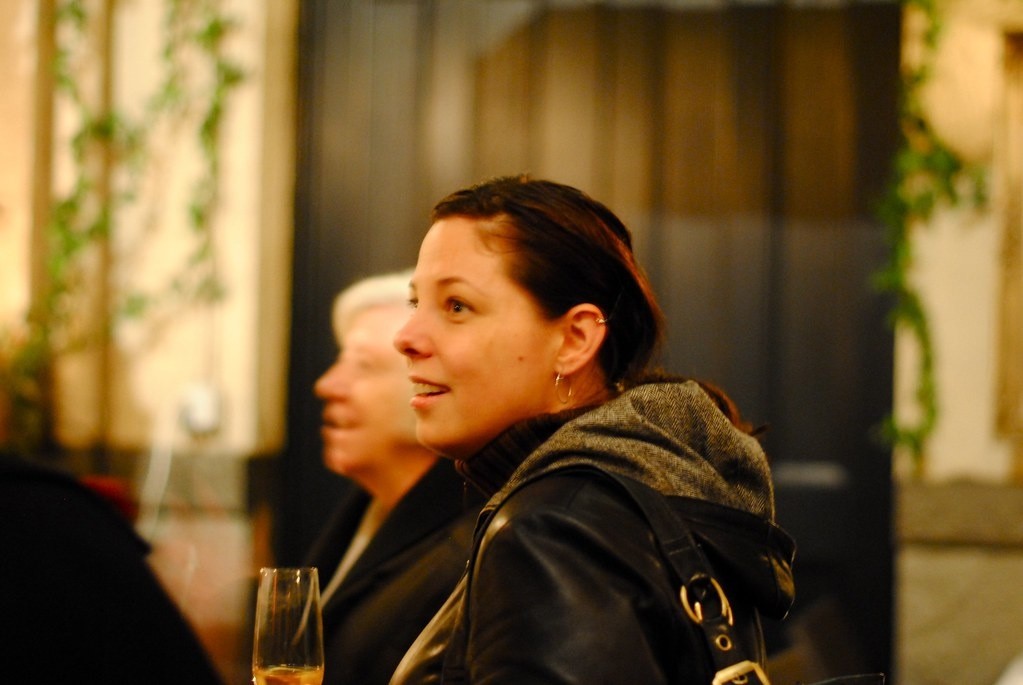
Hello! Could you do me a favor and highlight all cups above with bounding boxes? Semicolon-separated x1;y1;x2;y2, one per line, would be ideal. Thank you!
252;565;324;685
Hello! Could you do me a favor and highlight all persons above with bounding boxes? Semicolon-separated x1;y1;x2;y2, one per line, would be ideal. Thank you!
0;381;227;685
388;170;796;685
298;268;484;685
